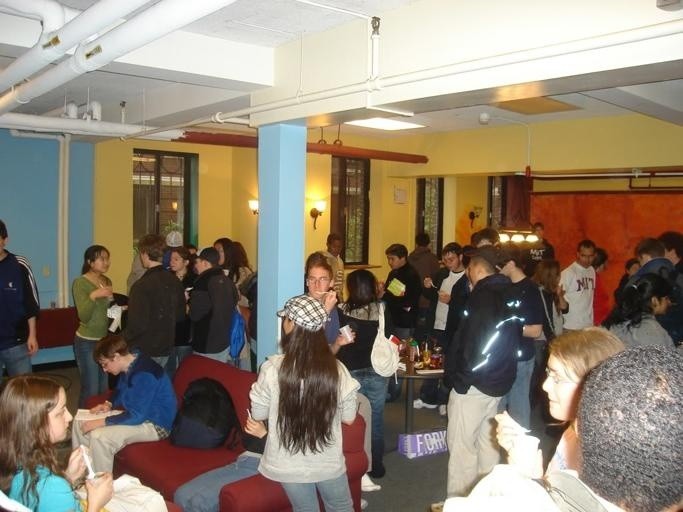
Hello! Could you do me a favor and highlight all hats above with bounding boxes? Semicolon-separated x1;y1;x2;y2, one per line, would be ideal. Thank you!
164;229;186;249
192;246;221;264
275;294;329;333
462;242;501;268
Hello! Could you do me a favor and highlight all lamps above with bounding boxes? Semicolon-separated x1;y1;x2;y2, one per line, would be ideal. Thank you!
246;197;260;216
309;201;328;230
466;204;484;230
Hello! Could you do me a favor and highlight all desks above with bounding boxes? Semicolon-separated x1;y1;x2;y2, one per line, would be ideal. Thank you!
388;346;447;453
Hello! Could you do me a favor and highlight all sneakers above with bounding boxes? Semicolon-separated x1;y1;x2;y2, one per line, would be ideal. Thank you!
359;474;383;492
413;397;440;410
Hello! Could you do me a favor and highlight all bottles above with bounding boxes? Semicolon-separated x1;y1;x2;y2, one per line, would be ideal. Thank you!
396;334;443;370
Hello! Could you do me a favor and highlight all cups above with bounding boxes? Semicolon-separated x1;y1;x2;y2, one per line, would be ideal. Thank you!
338;324;354;342
85;471;108;488
436;289;445;297
514;434;541;461
105;290;115;302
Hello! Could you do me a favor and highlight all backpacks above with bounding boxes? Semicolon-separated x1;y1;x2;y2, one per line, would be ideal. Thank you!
167;377;244;450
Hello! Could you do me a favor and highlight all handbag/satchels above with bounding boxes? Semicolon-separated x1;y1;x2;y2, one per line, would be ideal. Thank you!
369;303;402;377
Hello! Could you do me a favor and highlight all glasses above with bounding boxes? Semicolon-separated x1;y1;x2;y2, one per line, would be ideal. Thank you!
546;368;583;385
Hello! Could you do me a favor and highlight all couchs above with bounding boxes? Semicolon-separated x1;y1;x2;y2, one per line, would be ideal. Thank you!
78;353;370;510
